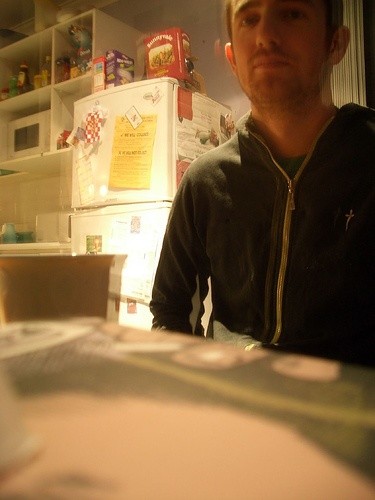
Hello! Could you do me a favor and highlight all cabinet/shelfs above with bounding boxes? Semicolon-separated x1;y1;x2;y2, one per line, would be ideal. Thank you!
0;8;147;170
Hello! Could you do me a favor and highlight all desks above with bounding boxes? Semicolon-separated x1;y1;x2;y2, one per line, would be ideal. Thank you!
0;320;375;500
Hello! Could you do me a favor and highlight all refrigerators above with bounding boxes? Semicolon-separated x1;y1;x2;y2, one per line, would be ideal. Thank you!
72;76;240;337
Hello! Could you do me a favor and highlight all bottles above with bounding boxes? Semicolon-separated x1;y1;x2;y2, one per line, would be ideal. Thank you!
9;75;18;97
18;65;29;94
42;56;50;87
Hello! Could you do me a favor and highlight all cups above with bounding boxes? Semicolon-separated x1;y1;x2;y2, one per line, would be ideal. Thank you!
3;224;16;244
34;75;41;89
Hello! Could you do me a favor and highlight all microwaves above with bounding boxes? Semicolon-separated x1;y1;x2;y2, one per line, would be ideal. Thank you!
7;109;49;159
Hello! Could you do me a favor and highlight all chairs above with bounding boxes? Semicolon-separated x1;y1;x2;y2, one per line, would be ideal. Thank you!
1;255;127;324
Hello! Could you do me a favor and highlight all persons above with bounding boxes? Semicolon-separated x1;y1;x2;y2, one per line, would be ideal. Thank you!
149;0;375;372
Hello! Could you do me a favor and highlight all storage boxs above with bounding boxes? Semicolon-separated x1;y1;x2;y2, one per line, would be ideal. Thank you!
146;27;195;84
94;48;135;92
